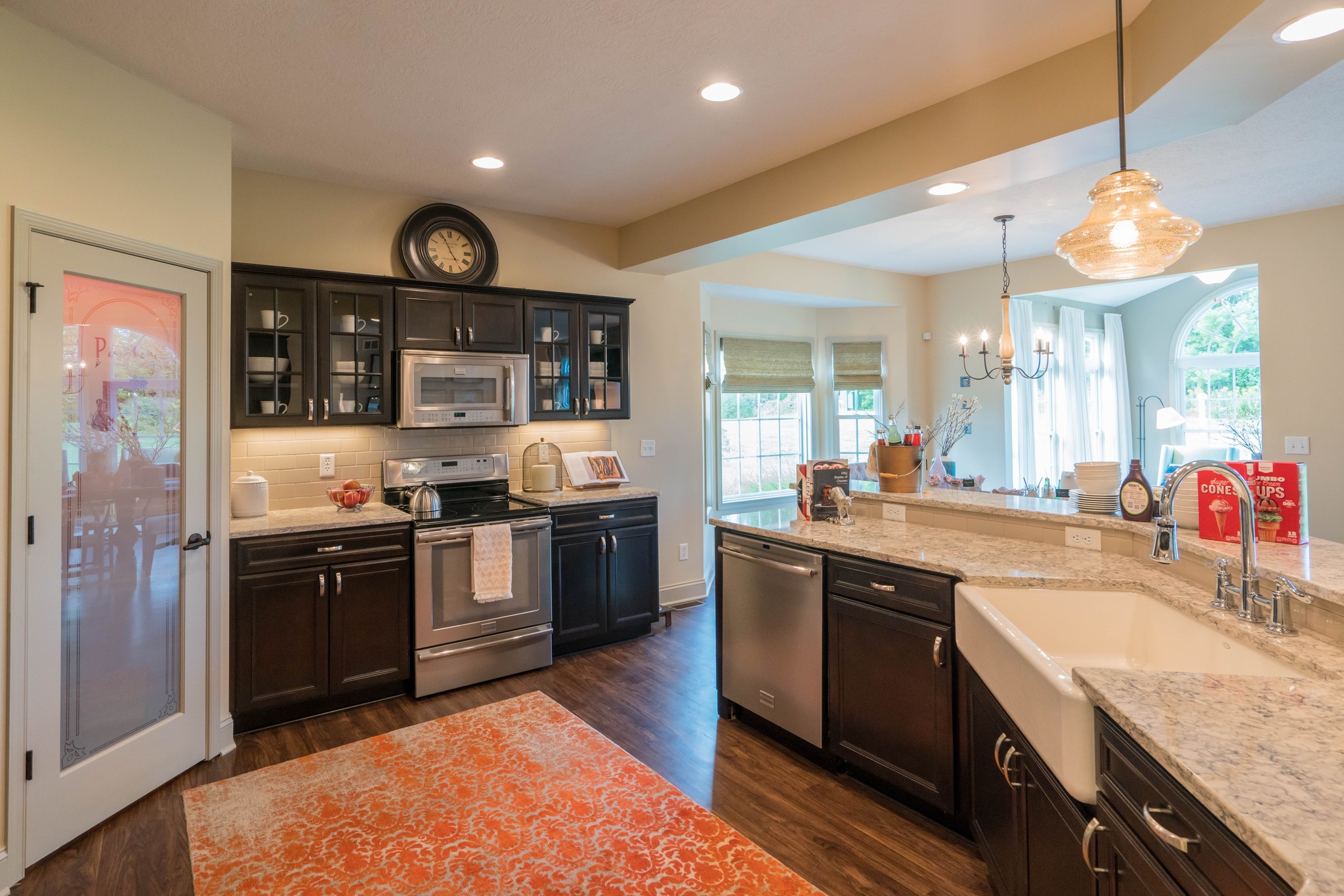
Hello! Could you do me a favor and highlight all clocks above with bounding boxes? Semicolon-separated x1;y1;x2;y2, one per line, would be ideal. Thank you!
397;202;499;288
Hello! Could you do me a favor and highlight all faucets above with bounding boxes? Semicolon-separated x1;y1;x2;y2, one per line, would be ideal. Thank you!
1149;460;1271;625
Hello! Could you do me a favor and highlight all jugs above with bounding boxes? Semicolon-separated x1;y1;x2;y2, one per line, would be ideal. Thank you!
1059;471;1075;501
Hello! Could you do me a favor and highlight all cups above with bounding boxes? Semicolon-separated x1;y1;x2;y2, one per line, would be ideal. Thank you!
922;481;933;494
590;399;605;409
541;399;560;410
260;401;288;414
925;481;942;489
540;327;559;343
589;330;607;344
1028;484;1039;498
964;486;975;492
1023;485;1037;498
948;484;961;490
974;484;982;492
338;400;362;413
1045;485;1056;500
961;484;964;490
1041;487;1053;499
340;315;366;333
261;311;289;329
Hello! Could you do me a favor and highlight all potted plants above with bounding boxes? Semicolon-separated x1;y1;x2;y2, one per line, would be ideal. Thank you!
1218;413;1264;460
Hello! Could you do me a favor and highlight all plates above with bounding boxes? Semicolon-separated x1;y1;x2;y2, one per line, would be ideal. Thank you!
1068;489;1121;514
814;504;837;507
990;489;1024;496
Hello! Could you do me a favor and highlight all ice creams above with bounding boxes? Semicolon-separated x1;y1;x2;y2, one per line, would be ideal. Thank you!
1256;495;1283;542
1208;495;1233;538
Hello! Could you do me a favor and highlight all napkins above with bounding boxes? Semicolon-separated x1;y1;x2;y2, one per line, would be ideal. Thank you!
927;472;942;486
969;474;986;485
992;486;1026;494
943;473;964;487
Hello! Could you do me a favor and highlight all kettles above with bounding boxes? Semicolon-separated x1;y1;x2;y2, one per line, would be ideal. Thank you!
404;481;442;512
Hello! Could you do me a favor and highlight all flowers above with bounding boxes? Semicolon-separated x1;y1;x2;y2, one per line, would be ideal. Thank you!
925;393;982;455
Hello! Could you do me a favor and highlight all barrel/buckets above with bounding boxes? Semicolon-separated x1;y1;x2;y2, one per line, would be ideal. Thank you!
870;441;926;493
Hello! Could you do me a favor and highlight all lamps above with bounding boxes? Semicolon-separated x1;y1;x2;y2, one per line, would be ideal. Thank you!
1135;395;1187;472
1191;266;1237;285
959;214;1053;385
1052;0;1203;281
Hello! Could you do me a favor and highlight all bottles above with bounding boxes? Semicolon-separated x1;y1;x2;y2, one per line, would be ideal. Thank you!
1120;459;1155;523
877;415;924;446
928;449;948;489
1044;478;1050;488
1056;479;1060;500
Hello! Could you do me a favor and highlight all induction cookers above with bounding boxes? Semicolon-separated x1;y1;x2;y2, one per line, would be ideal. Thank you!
383;453;550;529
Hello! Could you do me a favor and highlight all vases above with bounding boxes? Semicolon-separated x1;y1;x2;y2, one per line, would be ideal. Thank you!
927;450;947;488
932;455;956;478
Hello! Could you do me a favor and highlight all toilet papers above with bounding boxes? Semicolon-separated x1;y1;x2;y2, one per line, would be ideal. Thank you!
531;465;556;491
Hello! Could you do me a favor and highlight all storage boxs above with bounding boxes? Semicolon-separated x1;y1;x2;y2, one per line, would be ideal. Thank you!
1254;460;1309;545
1197;462;1259;545
870;442;924;493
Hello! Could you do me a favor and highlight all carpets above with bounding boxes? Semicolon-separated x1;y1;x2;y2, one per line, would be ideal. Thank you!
178;688;830;895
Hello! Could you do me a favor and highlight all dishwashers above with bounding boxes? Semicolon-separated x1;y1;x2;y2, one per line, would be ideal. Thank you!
715;531;827;753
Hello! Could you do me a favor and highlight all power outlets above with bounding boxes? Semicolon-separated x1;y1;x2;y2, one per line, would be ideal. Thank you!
882;503;907;522
640;440;655;457
679;543;688;561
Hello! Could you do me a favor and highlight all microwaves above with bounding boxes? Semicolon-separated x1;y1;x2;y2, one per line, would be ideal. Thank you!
378;349;530;429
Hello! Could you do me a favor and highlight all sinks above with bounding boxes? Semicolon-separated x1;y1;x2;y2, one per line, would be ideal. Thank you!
951;580;1310;806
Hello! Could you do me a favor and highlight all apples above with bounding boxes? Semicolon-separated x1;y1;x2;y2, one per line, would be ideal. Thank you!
328;479;371;507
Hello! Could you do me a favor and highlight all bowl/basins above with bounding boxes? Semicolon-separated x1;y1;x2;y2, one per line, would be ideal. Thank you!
536;361;561;386
1164;473;1200;531
248;357;290;383
336;369;366;384
1074;462;1122;494
589;361;608;389
336;361;365;370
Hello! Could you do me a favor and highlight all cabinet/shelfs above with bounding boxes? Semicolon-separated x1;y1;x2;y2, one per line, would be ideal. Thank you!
230;261;396;429
955;648;1095;895
230;501;412;739
524;288;636;422
705;506;964;833
394;275;525;353
510;484;662;659
1069;668;1343;896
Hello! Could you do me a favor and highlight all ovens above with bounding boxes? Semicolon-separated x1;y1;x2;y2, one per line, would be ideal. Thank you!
409;515;553;697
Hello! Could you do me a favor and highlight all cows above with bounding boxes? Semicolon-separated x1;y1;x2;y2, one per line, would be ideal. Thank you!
828;487;854;520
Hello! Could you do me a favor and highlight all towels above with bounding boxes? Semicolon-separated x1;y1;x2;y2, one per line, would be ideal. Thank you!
864;441;878;480
469;524;513;604
